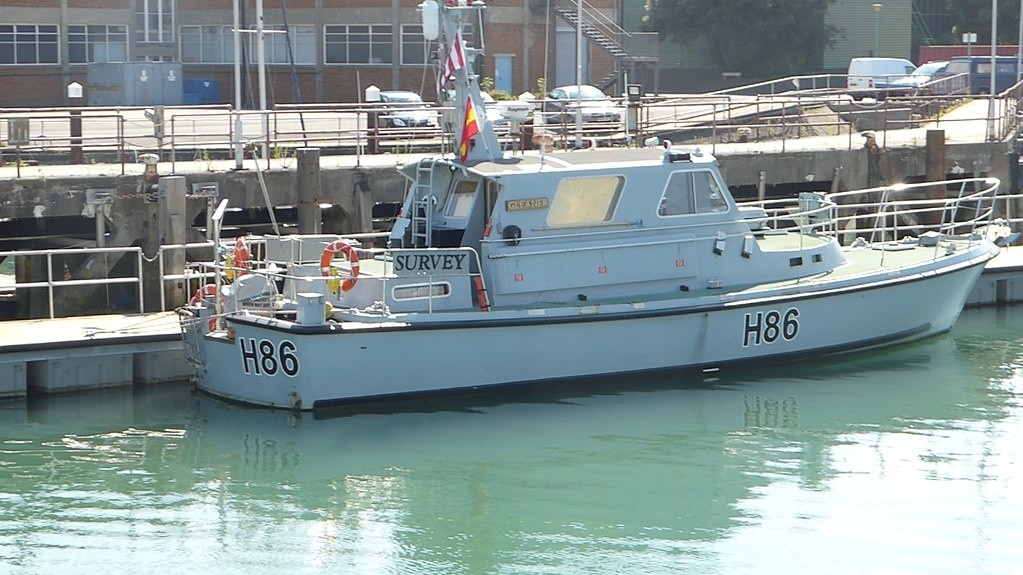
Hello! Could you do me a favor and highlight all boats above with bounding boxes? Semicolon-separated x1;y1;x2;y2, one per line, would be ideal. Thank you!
173;127;1002;412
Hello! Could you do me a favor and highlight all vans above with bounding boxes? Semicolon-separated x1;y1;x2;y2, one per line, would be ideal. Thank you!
846;58;918;102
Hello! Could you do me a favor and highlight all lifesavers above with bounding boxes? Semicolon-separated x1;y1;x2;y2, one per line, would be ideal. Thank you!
231;241;252;278
320;244;361;292
191;284;227;329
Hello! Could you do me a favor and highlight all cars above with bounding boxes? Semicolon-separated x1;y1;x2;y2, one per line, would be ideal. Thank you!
448;88;510;135
540;84;620;123
378;90;436;137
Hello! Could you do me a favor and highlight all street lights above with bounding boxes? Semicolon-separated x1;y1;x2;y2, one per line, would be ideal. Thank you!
872;3;882;58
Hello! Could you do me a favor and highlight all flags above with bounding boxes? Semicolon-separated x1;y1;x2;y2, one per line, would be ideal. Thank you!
460;95;480;163
438;31;465;93
